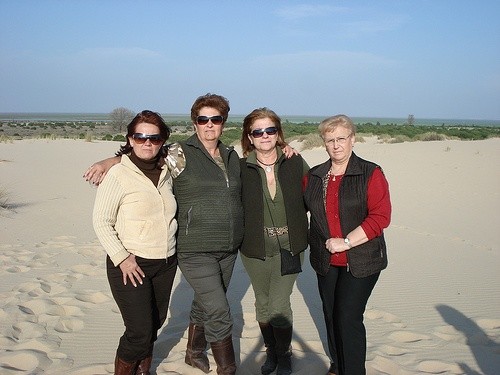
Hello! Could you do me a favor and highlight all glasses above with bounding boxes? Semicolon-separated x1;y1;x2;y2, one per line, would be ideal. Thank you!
132;133;164;145
250;127;278;138
325;132;354;146
197;115;226;125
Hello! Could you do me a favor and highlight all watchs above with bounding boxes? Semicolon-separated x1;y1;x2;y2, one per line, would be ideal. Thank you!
344;237;352;249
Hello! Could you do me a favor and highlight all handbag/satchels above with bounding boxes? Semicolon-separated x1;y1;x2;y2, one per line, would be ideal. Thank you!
279;248;302;277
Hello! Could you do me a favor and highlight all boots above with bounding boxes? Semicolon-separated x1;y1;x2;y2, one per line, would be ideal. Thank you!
273;326;292;375
210;333;236;375
258;321;278;375
185;322;213;374
114;350;137;375
134;356;151;375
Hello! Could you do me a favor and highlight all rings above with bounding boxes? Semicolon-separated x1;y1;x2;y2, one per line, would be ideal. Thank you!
90;165;94;169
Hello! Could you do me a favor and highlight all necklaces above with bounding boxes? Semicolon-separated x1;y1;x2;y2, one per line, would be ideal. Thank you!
331;164;347;176
255;156;277;165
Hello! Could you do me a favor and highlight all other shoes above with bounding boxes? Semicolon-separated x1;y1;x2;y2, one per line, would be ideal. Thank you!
326;362;337;375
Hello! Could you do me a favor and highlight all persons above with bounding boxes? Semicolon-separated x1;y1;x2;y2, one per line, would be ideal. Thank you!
92;110;177;375
302;115;391;375
83;94;298;375
238;108;312;375
